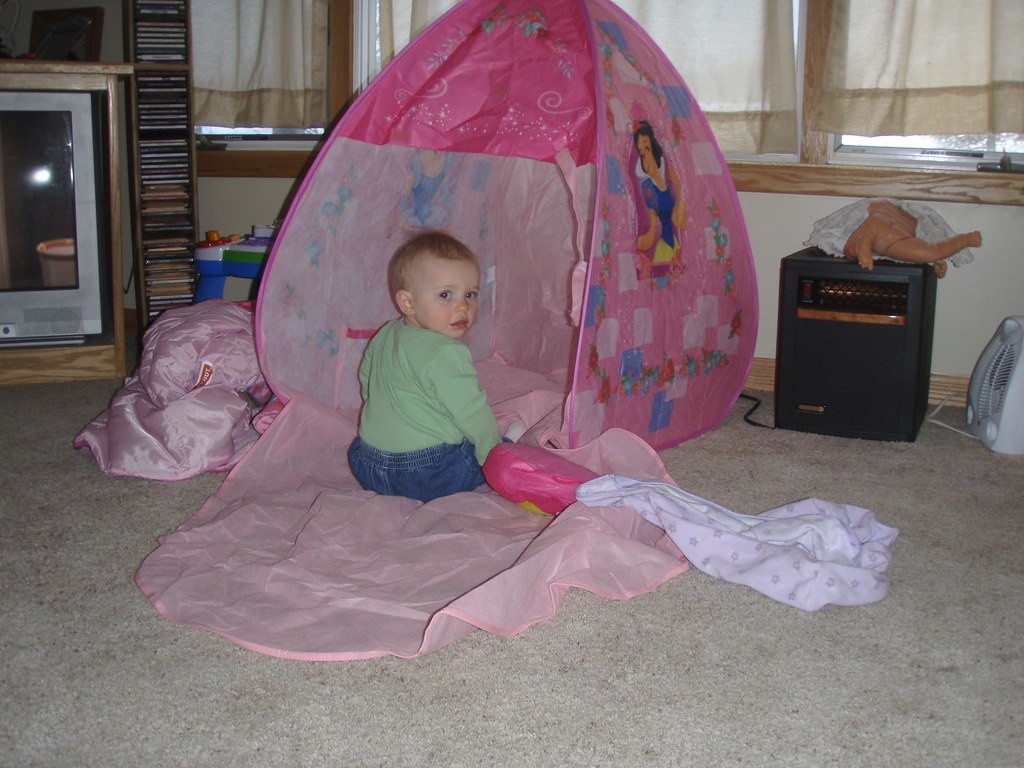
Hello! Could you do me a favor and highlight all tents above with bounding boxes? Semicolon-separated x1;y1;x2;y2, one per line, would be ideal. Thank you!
127;1;759;658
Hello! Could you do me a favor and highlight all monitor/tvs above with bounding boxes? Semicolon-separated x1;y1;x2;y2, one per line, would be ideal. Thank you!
0;90;106;348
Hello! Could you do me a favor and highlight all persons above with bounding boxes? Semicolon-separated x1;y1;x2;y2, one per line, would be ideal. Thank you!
347;231;527;502
809;195;982;279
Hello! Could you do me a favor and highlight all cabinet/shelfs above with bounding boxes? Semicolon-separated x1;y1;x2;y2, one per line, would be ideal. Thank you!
120;0;199;327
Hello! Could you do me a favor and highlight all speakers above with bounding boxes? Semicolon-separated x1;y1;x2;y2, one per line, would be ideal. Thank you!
773;246;939;442
